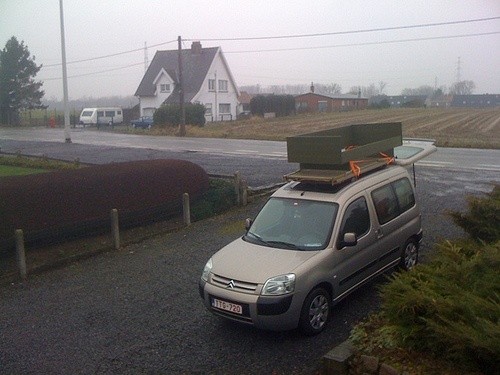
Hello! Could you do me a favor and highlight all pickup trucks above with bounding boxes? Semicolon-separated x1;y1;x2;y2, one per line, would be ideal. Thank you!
129;115;154;130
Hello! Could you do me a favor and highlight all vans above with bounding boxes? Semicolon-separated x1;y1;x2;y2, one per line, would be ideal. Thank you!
199;166;423;337
